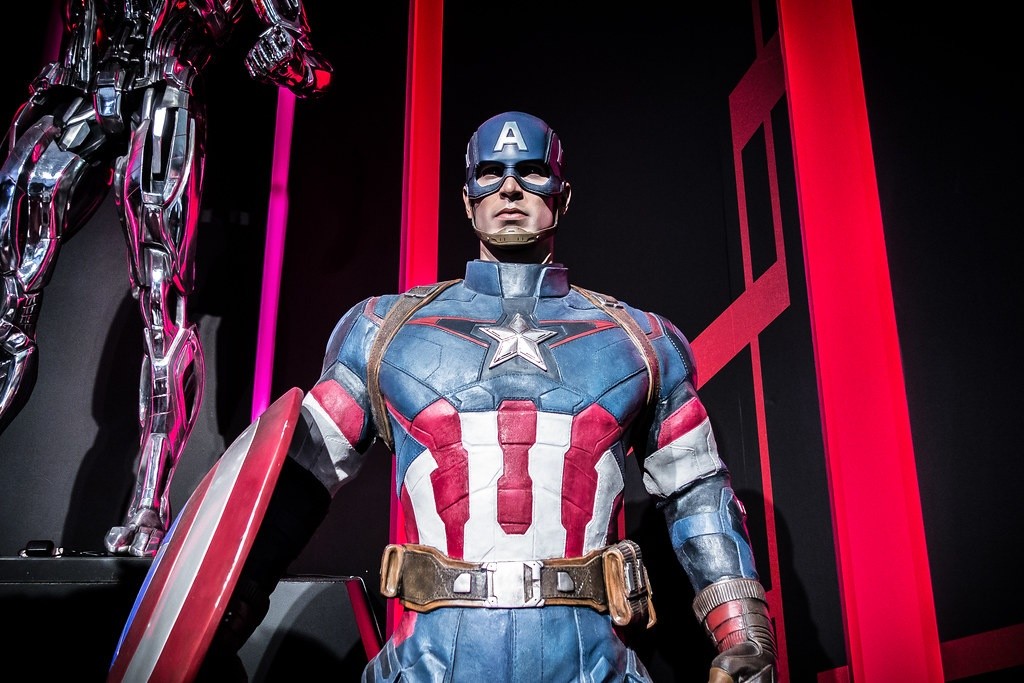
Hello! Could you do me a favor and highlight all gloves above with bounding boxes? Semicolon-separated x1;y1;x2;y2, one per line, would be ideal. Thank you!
692;579;778;683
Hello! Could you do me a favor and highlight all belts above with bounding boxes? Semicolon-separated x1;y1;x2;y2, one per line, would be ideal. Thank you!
397;545;609;615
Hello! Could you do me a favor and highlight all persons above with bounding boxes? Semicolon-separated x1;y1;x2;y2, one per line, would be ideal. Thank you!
204;110;776;682
0;0;339;557
